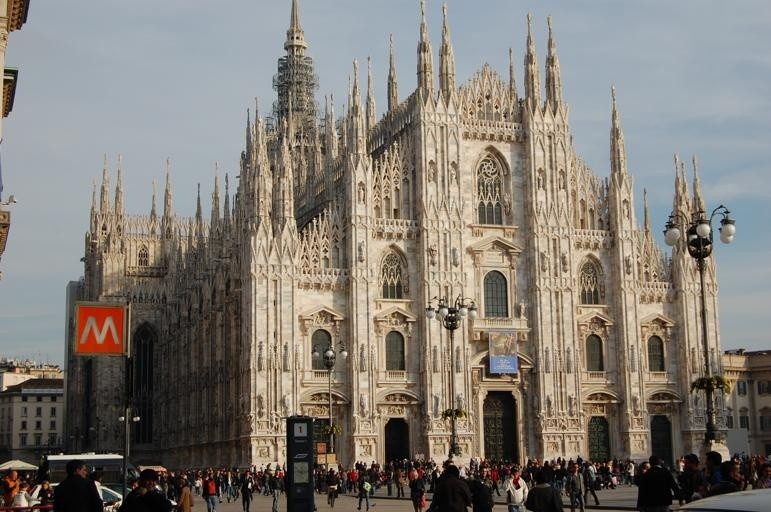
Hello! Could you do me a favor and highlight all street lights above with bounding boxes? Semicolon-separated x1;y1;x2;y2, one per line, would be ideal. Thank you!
423;295;477;452
114;403;141;462
664;205;736;443
310;340;352;452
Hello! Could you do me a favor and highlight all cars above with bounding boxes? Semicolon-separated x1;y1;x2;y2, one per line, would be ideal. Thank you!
28;483;123;511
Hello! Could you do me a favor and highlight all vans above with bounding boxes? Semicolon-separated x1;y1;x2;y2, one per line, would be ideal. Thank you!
41;452;139;484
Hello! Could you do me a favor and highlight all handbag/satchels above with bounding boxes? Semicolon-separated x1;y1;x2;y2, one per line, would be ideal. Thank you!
362;481;372;493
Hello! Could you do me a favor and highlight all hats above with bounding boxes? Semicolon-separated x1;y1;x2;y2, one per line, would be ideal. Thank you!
511;466;520;472
140;469;160;483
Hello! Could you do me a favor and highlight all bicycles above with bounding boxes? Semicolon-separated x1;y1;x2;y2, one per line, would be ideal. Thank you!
328;488;336;508
